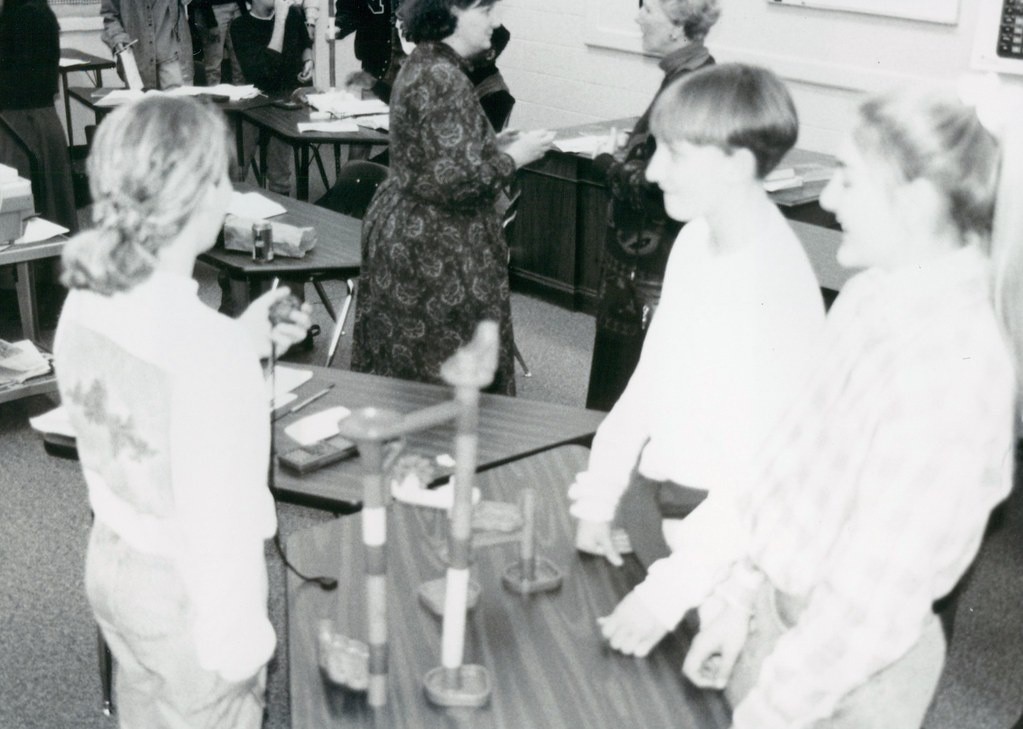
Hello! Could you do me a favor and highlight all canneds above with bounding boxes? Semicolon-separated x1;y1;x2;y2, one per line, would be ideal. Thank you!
252;222;273;261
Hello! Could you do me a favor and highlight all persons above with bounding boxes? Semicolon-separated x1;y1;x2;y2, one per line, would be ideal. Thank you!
587;0;725;411
680;68;1018;729
52;90;314;729
349;1;557;399
187;0;321;195
0;0;79;341
315;19;517;222
100;0;197;96
328;0;409;109
570;64;825;662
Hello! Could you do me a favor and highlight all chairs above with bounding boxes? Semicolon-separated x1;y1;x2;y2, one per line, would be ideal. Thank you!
269;158;390;366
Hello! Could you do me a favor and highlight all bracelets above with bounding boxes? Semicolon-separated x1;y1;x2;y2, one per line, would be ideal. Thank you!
303;59;314;69
306;22;315;27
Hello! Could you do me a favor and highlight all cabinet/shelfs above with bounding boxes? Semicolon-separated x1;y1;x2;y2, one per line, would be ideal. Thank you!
0;216;68;404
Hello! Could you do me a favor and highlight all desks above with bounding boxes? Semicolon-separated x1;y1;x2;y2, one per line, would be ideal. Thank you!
199;182;364;317
58;46;389;203
506;114;847;331
790;218;871;306
42;360;738;728
